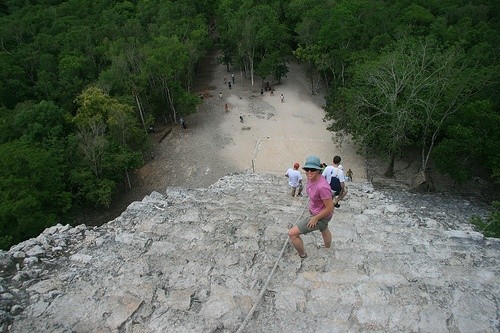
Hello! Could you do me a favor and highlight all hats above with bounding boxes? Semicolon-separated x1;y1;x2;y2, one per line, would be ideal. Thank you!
294;162;299;168
302;155;324;170
338;165;343;170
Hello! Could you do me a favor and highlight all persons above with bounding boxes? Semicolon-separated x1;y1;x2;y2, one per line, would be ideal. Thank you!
346;168;354;182
321;155;348;208
285;163;304;197
150;73;285;134
288;155;335;259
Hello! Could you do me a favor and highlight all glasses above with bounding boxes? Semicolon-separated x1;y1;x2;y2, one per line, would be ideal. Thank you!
304;169;317;172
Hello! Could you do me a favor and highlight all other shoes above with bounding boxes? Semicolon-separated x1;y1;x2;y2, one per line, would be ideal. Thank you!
298;192;303;197
292;195;296;197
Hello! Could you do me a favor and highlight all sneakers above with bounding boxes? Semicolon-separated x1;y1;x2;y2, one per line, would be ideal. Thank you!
316;243;325;249
334;202;340;207
295;252;307;259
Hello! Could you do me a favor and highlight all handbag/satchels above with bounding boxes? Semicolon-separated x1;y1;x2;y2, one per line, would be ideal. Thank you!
331;169;341;192
